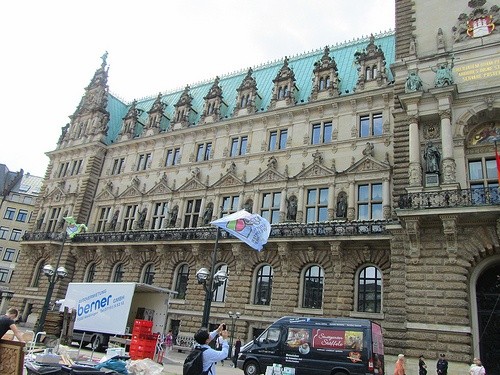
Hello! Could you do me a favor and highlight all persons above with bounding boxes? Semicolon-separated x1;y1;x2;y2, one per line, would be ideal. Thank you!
106;215;118;231
394;354;405;375
407;73;422;91
469;358;486;375
0;308;26;343
286;196;297;220
136;210;145;228
194;323;229;375
430;57;454;87
337;194;347;217
436;353;448;375
424;140;440;174
166;330;173;357
166;207;178;228
419;355;428;375
203;205;213;224
215;332;224;367
243;201;251;213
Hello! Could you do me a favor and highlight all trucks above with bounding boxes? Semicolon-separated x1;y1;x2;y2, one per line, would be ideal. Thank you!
50;282;178;356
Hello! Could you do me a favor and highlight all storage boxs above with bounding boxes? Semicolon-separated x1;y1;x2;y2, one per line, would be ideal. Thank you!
129;320;158;360
105;347;126;358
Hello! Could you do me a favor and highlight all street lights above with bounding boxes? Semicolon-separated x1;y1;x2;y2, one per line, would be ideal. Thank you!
32;232;69;343
195;228;228;326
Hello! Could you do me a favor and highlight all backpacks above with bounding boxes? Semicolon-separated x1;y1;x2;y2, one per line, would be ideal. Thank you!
183;346;213;375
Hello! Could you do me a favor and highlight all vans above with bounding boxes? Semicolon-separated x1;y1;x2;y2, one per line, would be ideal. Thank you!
232;314;385;375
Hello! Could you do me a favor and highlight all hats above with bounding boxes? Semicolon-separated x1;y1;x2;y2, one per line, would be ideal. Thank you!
440;354;445;357
419;355;424;358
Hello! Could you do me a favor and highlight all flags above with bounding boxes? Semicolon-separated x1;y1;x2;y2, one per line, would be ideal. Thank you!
64;217;88;237
213;209;270;251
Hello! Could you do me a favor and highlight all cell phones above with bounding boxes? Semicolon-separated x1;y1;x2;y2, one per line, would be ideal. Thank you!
223;325;226;330
